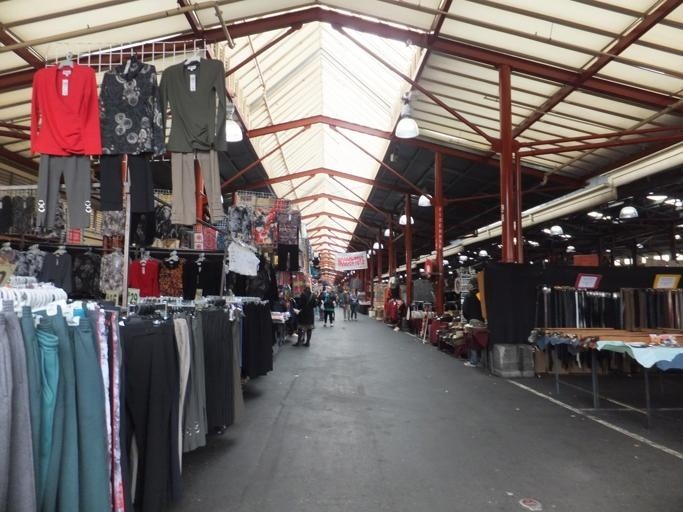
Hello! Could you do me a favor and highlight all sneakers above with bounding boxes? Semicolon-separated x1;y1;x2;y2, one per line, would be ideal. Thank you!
292;317;358;347
464;361;478;366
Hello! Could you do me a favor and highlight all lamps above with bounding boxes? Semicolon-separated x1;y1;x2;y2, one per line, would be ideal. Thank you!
217;90;244;143
393;90;423;140
340;183;682;283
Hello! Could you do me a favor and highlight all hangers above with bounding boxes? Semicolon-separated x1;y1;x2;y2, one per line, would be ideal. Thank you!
535;284;683;305
0;53;321;338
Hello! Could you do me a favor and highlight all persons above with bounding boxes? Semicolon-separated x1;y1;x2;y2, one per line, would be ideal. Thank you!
271;279;360;347
462;277;485;368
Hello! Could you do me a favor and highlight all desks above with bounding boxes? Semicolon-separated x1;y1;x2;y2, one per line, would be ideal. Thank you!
529;327;683;429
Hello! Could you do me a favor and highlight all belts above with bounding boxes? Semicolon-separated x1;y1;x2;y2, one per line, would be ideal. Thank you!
534;286;682;374
